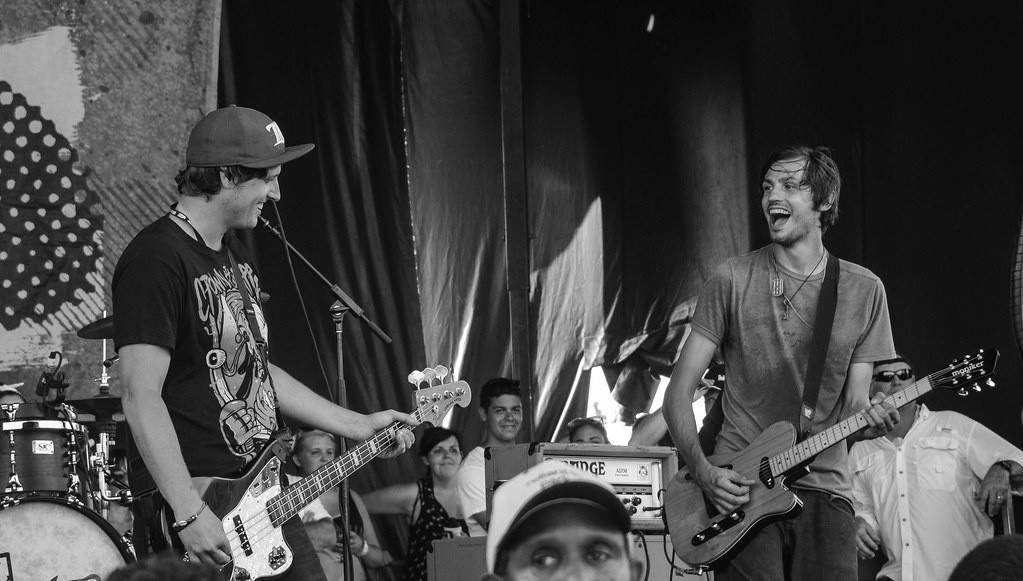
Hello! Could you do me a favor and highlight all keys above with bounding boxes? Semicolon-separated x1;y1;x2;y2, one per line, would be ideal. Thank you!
782;299;791;320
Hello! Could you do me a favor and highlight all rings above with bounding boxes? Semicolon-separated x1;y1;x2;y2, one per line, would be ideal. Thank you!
997;496;1003;499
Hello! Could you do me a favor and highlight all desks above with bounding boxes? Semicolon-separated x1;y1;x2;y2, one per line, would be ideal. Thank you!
427;533;715;580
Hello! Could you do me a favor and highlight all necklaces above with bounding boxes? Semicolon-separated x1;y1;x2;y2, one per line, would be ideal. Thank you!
771;242;827;330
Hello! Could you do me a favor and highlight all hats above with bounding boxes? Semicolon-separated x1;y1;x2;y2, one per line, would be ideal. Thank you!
185;103;316;169
874;353;915;368
484;460;633;575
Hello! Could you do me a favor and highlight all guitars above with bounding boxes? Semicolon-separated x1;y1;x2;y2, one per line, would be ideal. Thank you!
162;363;474;581
663;346;1002;570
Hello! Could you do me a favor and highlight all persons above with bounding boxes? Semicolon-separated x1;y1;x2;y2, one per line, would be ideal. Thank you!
481;459;645;581
455;378;523;537
110;106;417;566
661;145;898;581
558;364;724;471
284;427;470;581
848;355;1023;581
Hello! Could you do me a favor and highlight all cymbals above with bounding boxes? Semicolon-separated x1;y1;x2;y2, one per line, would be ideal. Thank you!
77;314;113;340
63;393;124;414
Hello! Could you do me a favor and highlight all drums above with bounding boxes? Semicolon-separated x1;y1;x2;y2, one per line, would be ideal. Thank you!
0;492;137;581
1;419;90;507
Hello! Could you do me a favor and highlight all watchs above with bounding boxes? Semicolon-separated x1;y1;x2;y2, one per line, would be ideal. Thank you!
992;461;1012;475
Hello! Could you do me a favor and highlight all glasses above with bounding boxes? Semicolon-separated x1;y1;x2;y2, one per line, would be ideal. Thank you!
567;416;605;431
872;368;914;382
485;379;521;396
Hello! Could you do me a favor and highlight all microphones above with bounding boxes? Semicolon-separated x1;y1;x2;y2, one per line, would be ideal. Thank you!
35;352;56;397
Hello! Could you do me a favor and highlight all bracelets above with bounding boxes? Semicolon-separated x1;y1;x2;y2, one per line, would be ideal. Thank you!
171;502;208;533
355;536;368;558
